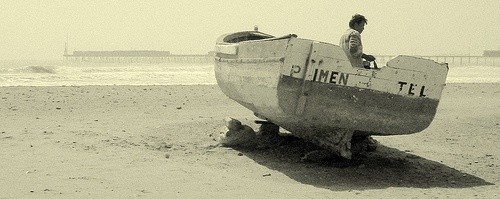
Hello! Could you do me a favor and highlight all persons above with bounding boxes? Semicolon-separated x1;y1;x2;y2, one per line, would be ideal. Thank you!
339;14;375;70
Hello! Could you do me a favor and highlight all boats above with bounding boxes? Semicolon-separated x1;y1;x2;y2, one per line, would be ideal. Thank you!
214;27;449;160
